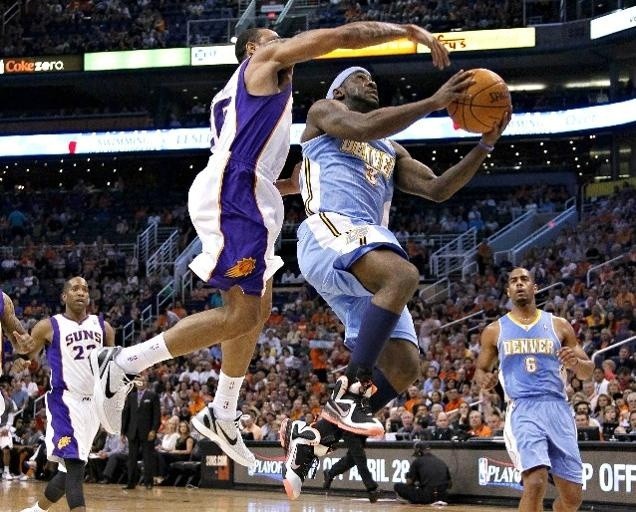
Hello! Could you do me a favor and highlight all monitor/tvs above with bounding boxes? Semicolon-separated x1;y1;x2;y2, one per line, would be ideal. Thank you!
575;426;600;440
615;432;635;440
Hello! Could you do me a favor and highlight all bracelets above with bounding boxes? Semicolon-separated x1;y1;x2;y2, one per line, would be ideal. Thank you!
479;138;495;153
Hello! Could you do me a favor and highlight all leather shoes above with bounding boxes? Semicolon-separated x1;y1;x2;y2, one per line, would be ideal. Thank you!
83;474;199;489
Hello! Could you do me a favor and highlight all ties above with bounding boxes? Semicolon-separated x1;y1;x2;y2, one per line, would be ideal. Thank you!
138;392;142;406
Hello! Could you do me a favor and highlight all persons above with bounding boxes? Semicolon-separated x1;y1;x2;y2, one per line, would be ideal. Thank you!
87;17;452;468
2;183;636;510
0;1;636;64
277;62;513;499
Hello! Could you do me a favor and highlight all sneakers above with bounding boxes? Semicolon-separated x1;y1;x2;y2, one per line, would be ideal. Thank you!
190;401;255;468
1;459;37;481
280;418;333;501
369;488;380;504
87;346;141;437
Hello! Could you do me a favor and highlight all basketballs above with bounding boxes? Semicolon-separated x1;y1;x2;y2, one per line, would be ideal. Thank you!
447;69;510;133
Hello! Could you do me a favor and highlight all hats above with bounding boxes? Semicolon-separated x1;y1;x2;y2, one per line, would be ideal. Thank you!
413;443;429;456
